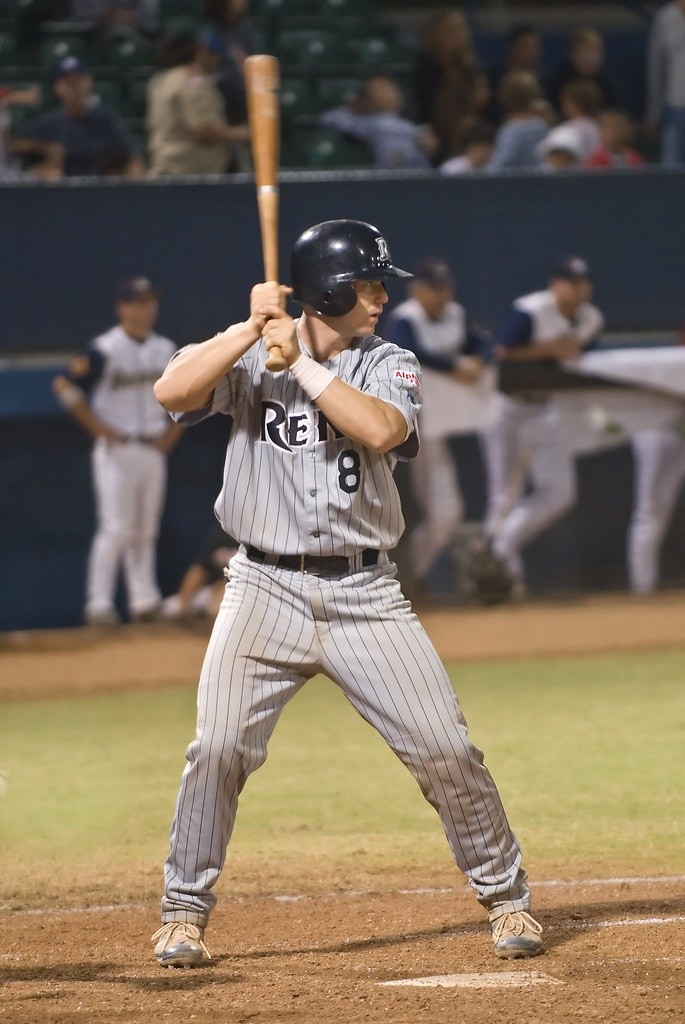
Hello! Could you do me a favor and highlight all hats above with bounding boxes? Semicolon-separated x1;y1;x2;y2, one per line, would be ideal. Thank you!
532;125;585;161
412;254;453;288
47;55;94;85
552;253;602;279
120;275;157;299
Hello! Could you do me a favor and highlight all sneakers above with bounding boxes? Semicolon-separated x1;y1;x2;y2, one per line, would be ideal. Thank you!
151;922;212;968
490;909;544;959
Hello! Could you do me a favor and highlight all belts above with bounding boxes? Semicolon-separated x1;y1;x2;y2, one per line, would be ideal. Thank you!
247;545;380;577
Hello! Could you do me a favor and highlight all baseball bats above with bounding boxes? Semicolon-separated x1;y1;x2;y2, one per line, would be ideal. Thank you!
239;50;291;374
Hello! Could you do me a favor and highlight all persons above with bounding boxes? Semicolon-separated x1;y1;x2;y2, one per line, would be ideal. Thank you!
51;278;187;622
156;257;609;618
0;0;685;181
149;219;544;969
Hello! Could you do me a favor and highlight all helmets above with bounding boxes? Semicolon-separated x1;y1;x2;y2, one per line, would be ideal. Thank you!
289;218;414;317
